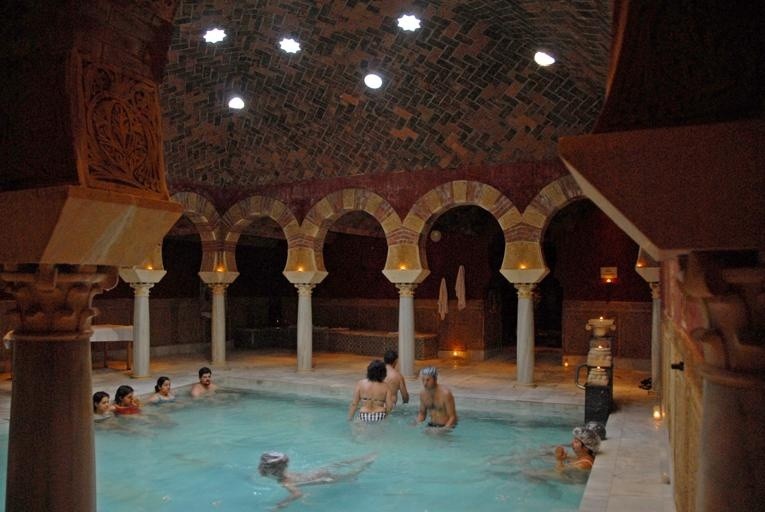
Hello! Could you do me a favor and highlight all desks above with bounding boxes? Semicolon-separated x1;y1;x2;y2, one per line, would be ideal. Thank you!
2;324;133;370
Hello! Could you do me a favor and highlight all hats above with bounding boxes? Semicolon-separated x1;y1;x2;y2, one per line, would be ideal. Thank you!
572;426;601;453
260;451;289;479
585;421;606;439
420;367;438;377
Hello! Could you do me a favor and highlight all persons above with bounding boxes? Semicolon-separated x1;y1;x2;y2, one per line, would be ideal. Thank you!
479;420;606;485
93;367;219;440
346;350;458;440
258;447;380;510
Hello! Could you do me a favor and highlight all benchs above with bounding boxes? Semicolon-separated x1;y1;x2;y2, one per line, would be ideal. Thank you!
231;322;440;361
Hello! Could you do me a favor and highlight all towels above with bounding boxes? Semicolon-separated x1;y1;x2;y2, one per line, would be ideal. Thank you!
436;263;467;321
